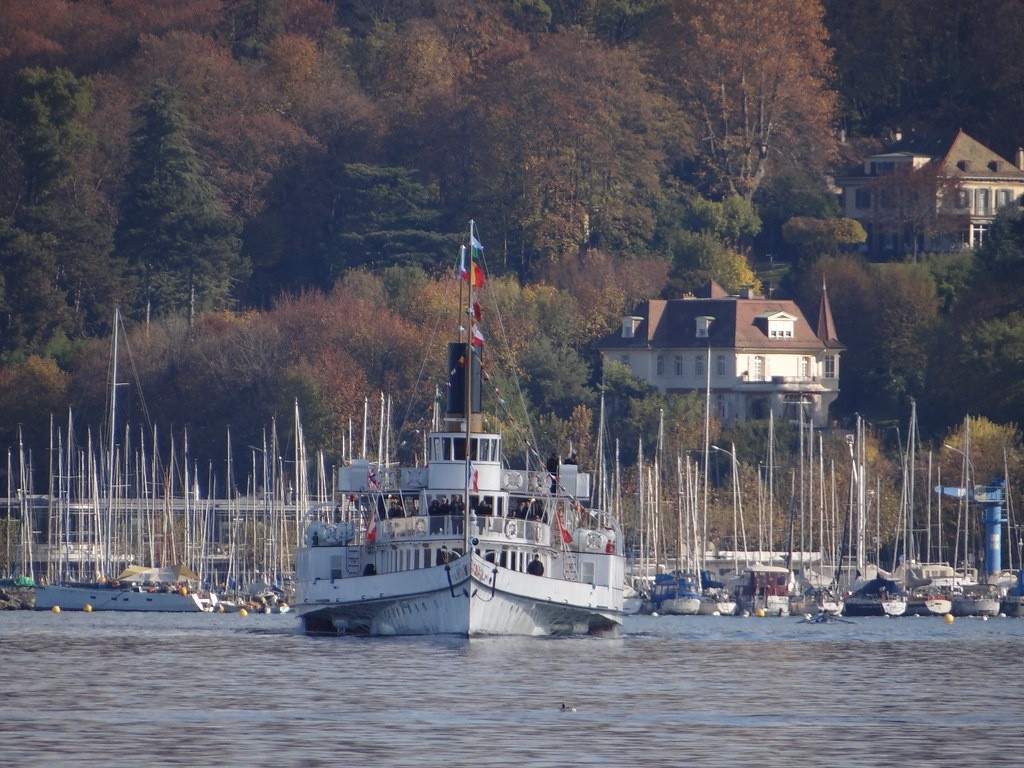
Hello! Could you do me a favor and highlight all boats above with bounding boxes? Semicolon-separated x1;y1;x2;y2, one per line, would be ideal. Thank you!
296;219;624;636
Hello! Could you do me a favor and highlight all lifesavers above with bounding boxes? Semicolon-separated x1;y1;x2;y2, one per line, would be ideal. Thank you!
415;517;428;537
536;523;543;542
585;531;604;549
404;467;424;487
533;474;553;491
504;521;518;539
504;471;523;491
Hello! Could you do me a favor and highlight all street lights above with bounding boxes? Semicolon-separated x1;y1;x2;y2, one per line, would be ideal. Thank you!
711;444;745;548
944;444;976;568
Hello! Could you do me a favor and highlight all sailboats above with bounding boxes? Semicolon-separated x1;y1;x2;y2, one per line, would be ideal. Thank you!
0;308;1023;616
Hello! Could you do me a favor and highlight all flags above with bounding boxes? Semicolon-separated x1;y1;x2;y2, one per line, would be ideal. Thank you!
367;509;376;542
556;510;572;543
367;467;378;489
458;266;469;281
469;237;484;348
465;458;478;492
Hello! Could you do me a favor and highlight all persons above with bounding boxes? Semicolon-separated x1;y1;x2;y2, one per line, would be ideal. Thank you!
547;451;559;496
527;554;544;576
388;495;538;535
816;609;829;623
564;453;578;465
